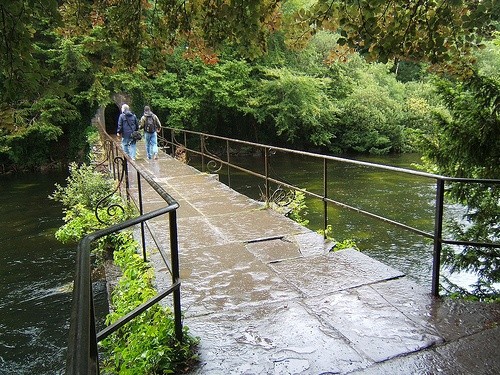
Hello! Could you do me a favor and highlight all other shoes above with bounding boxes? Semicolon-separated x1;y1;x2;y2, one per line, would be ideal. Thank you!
154;154;159;159
128;154;135;161
147;154;152;159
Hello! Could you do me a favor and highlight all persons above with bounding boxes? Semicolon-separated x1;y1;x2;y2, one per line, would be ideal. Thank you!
117;103;140;161
138;105;163;159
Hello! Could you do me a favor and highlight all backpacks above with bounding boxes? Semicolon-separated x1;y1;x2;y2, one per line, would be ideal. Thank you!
144;113;156;133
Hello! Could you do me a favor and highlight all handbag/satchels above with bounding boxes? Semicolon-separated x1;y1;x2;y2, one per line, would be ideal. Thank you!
131;131;142;140
